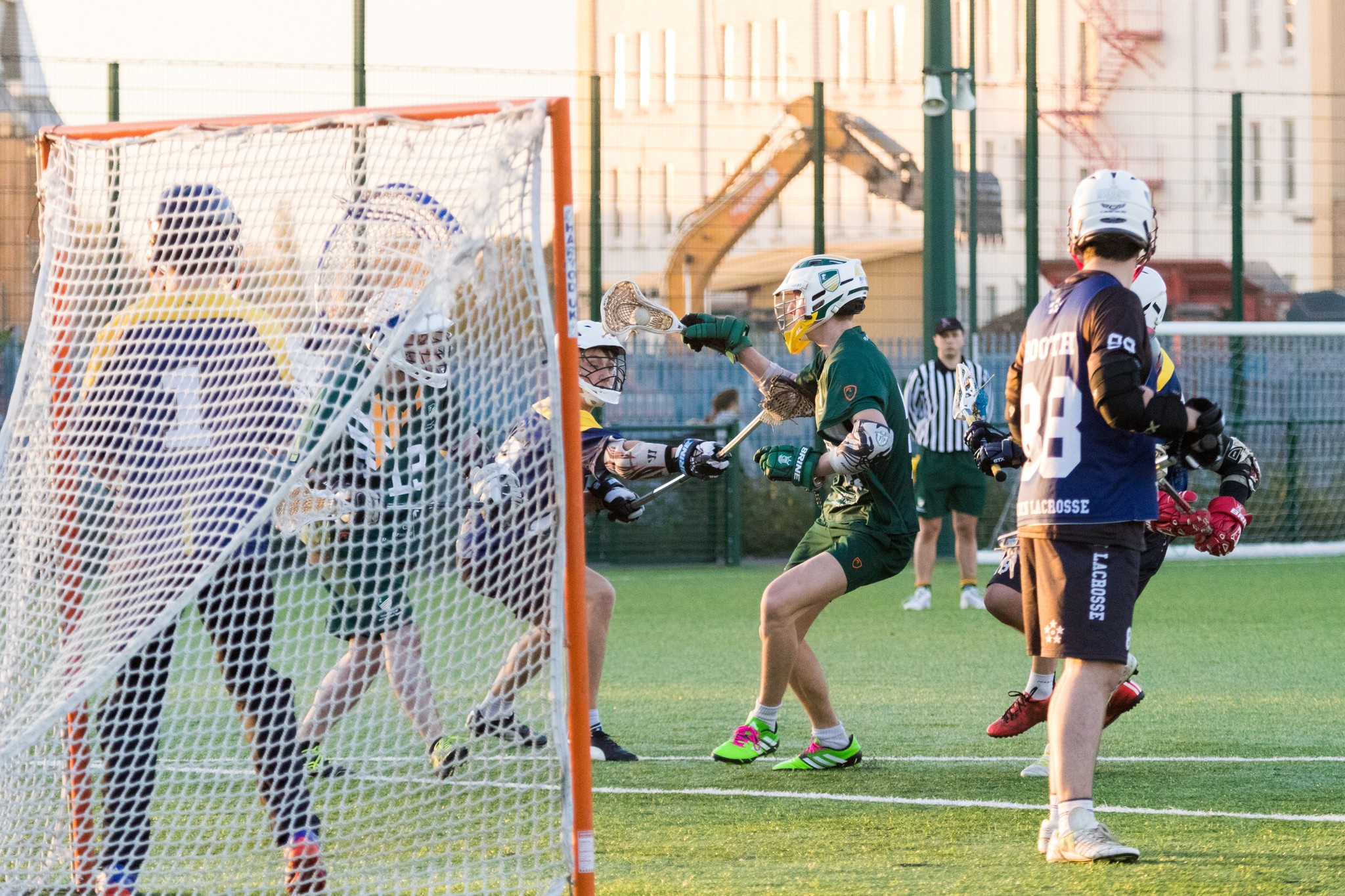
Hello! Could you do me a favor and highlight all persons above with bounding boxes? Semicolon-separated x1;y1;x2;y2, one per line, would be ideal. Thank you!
705;389;757;565
74;185;328;896
905;316;993;612
272;286;523;786
1003;167;1226;862
457;320;731;762
680;254;918;770
964;265;1260;778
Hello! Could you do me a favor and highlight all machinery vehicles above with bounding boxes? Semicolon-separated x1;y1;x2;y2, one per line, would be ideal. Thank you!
663;95;1006;331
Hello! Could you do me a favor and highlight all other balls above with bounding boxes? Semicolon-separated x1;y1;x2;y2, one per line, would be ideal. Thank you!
630;306;651;326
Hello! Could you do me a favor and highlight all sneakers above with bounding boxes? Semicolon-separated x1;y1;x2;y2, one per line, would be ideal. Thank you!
284;828;327;894
465;703;548;748
300;741;357;779
772;734;863;770
959;586;986;610
711;717;780;762
429;732;468;779
902;587;931;612
567;730;639;762
988;652;1144;864
94;862;140;896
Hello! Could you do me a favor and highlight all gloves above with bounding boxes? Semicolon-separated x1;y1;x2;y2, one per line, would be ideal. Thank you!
1145;490;1212;538
1163;397;1227;470
974;438;1028;477
1193;495;1253;558
678;313;753;365
330;480;388;528
963;420;1013;454
466;463;529;531
588;434;823;525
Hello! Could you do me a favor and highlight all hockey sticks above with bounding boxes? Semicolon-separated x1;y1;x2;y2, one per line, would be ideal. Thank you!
303;181;464;348
273;479;485;534
952;363;1006;483
1155;442;1196;514
606;375;816;522
600;279;727;351
997;454;1178;554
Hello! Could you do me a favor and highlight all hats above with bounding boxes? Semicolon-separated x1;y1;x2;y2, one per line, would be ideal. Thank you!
936;318;963;335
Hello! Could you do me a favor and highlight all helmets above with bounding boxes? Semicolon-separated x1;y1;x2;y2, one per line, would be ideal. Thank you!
1129;264;1168;331
771;254;869;355
151;182;242;272
553;320;627;406
1067;169;1154;253
360;286;455;389
